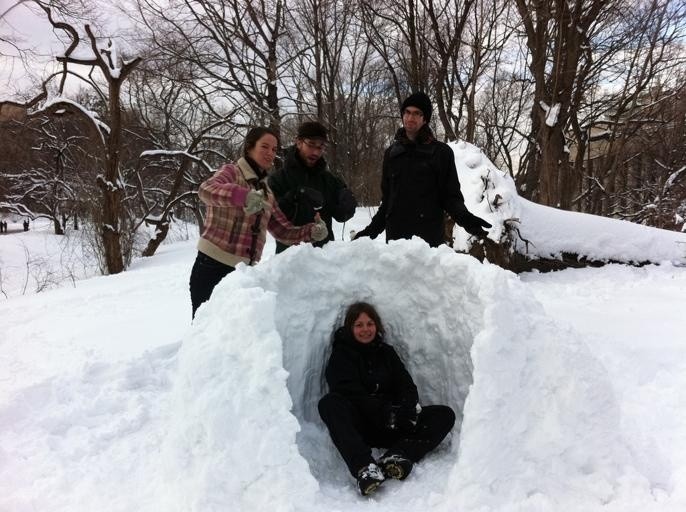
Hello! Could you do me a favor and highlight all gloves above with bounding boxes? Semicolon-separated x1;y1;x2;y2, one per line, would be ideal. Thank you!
462;215;493;239
352;226;377;240
246;188;273;213
310;216;329;242
338;189;353;209
306;188;324;209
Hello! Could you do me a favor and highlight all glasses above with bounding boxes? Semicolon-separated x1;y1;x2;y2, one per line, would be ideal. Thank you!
403;110;423;117
302;140;325;152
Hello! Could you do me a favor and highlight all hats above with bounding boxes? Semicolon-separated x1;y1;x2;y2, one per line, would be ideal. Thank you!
297;122;332;144
400;91;432;123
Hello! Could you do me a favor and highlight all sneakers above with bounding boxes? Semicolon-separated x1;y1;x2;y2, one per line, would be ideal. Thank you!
357;463;386;495
375;454;412;480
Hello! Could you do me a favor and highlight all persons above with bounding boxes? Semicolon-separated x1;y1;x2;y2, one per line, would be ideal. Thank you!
318;303;455;497
349;93;492;249
189;126;328;323
264;120;356;255
